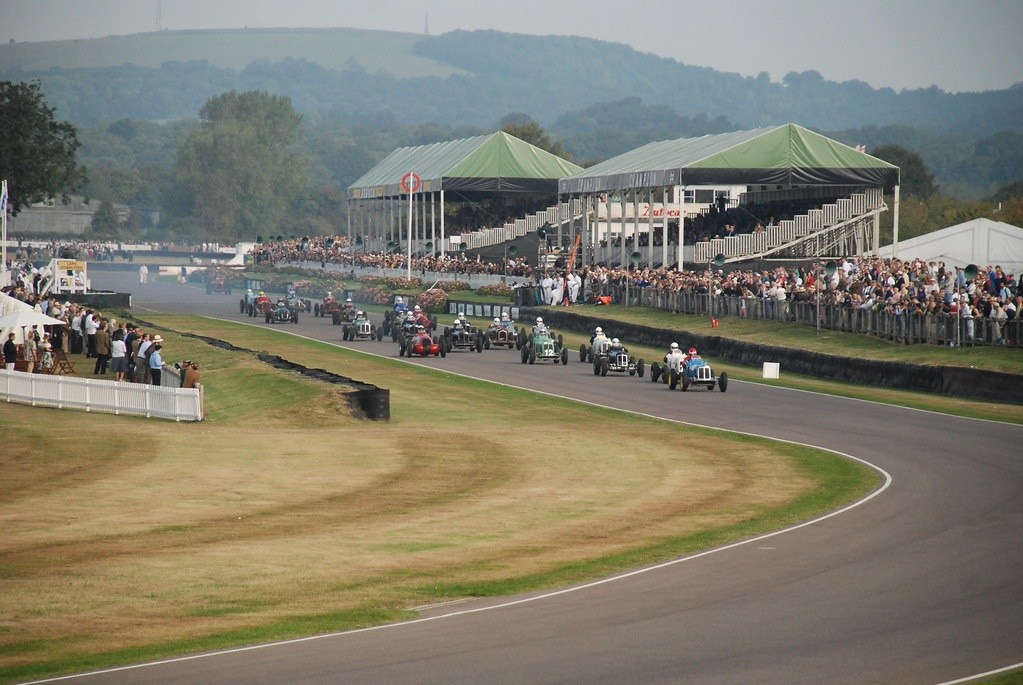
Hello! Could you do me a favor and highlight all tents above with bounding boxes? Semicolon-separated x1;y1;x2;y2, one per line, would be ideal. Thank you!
347;130;584;256
558;123;901;272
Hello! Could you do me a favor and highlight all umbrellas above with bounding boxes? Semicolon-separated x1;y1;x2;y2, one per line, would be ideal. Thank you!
0;309;66;341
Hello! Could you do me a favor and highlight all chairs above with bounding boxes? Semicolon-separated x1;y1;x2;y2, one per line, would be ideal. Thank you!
55;348;76;375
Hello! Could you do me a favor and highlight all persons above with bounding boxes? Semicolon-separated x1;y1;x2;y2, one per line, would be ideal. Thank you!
663;342;678;364
532;317;546;335
249;234;533;277
245;288;295;311
590;326;603;344
138;263;149;284
606;338;628;353
344;298;356;310
323;291;335;305
395;305;507;331
180;264;187;284
583;203;1023;347
353;311;362;324
393;297;405;315
682;348;697;368
0;234;231;388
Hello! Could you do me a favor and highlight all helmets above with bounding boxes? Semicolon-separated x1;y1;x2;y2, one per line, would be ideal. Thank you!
458;313;465;320
259;291;265;297
346;298;352;306
414;305;420;312
670;342;678;351
689;348;696;353
407;311;413;318
397;297;403;303
493;318;501;325
612;338;619;346
418;324;425;333
536;317;543;325
357;311;363;318
454;319;461;328
595;326;603;334
247;289;252;295
502;312;507;321
328;292;332;297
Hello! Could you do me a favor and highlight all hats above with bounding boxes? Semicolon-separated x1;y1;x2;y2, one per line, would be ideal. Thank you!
65;301;71;306
55;302;60;305
153;335;163;343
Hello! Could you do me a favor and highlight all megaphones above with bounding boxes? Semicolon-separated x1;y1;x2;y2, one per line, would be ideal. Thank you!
630;251;642;262
961;264;978;280
457;242;467;252
422;241;433;252
821;261;836;274
386;241;395;249
505;244;519;256
710;253;725;266
255;235;362;251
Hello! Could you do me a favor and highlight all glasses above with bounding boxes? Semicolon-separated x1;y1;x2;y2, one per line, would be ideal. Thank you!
419;329;424;331
